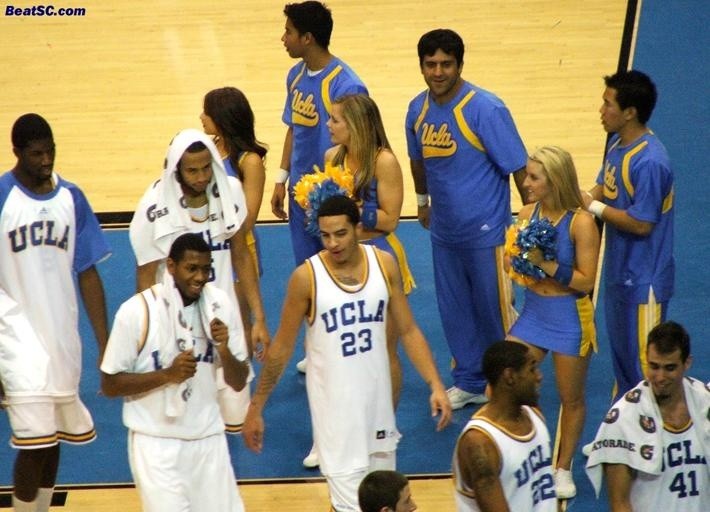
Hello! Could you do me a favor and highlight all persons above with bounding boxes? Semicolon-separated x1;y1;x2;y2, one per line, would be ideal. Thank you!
404;30;532;412
267;0;370;372
1;114;109;512
358;471;417;511
98;233;254;511
199;89;264;329
243;195;452;511
454;340;562;512
127;126;272;364
605;320;709;512
585;71;677;406
499;148;601;502
292;92;416;467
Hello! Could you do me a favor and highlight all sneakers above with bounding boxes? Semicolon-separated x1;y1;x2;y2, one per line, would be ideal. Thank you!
296;358;307;373
437;385;489;410
556;457;577;499
303;444;319;468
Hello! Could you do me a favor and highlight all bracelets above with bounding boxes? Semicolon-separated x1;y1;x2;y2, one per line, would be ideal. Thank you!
274;165;288;186
361;209;379;230
553;263;573;289
587;200;608;220
413;191;431;209
